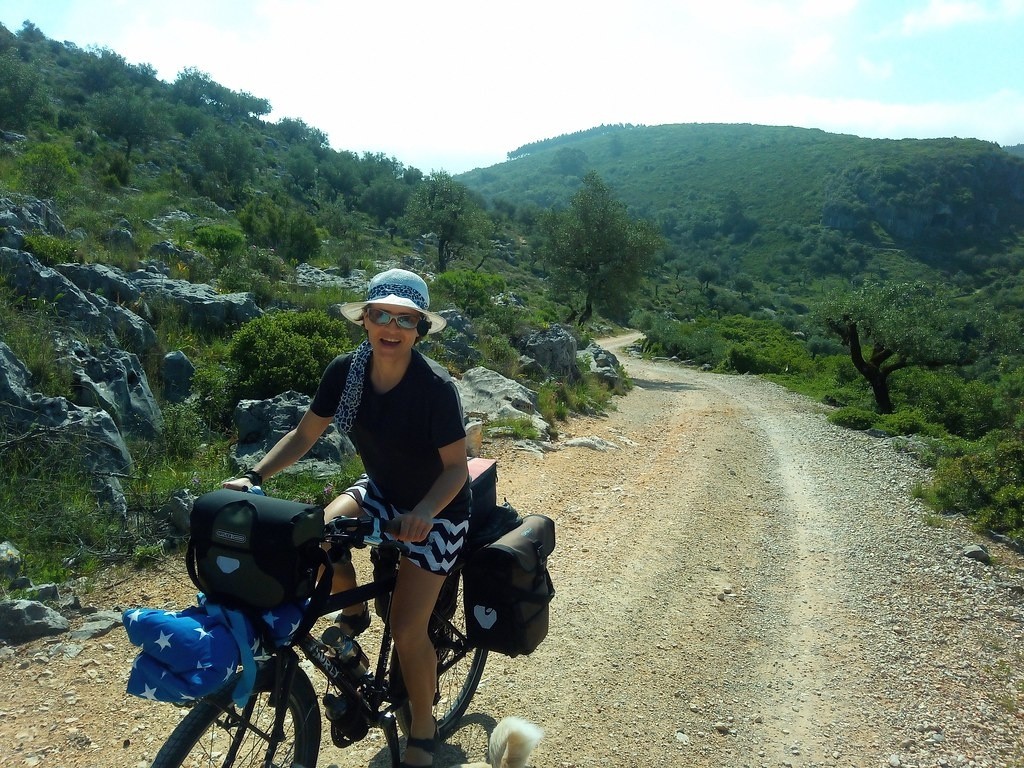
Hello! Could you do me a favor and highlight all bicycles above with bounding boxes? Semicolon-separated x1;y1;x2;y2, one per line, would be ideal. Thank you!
146;460;557;768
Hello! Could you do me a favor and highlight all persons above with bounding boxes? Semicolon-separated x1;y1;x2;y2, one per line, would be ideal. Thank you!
220;267;473;768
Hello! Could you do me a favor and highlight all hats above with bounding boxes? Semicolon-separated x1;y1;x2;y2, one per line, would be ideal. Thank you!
340;267;447;334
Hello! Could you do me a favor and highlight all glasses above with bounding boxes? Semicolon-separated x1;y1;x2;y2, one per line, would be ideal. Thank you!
366;307;423;329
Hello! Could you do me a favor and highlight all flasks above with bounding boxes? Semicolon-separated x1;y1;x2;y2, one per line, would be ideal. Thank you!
322;626;369;681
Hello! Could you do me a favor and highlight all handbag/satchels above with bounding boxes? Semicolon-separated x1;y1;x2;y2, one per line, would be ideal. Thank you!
461;512;556;657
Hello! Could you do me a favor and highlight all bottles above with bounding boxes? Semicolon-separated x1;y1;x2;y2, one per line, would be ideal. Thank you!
323;694;368;742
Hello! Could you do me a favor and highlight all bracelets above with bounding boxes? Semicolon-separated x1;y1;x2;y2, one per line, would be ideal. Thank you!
240;470;263;486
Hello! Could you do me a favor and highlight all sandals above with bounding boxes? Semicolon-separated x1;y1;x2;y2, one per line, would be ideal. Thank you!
318;603;373;647
401;714;437;768
